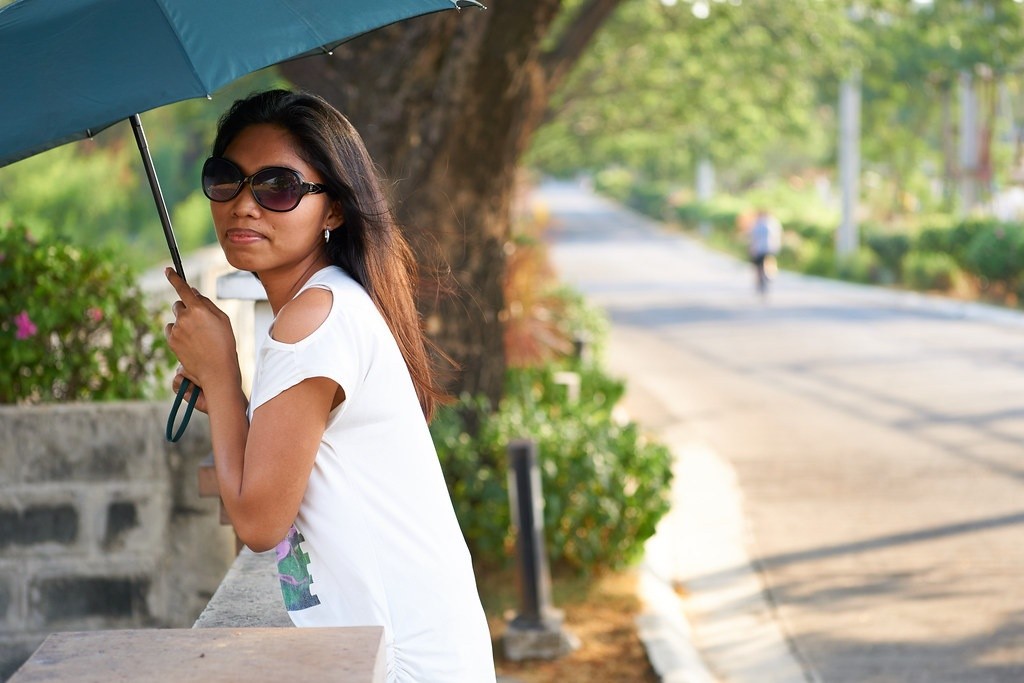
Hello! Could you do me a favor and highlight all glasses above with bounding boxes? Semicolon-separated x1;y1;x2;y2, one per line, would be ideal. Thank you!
201;158;337;212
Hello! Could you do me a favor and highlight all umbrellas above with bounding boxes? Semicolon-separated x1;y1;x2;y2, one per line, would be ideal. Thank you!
0;0;487;281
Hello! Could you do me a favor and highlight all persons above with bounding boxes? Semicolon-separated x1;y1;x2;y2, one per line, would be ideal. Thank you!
750;208;781;291
165;88;498;683
